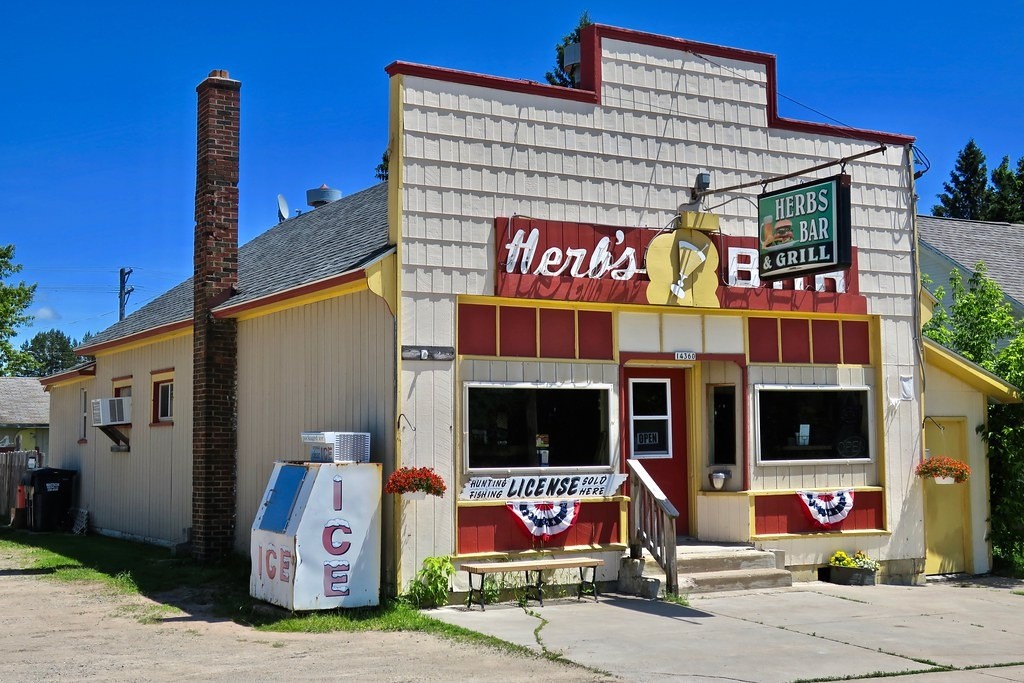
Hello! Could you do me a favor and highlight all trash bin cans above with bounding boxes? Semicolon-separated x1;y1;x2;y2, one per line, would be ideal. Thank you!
27;466;78;531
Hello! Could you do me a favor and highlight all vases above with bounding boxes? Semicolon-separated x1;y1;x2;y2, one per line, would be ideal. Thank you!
935;476;955;485
817;566;876;585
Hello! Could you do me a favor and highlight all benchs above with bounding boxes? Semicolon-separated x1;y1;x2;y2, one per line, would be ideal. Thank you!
460;557;604;612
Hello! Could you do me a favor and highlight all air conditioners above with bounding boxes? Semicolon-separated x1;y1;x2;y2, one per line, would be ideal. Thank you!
91;397;132;426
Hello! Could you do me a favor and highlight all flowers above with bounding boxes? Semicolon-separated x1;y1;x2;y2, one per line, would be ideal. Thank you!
914;456;971;483
830;550;880;572
